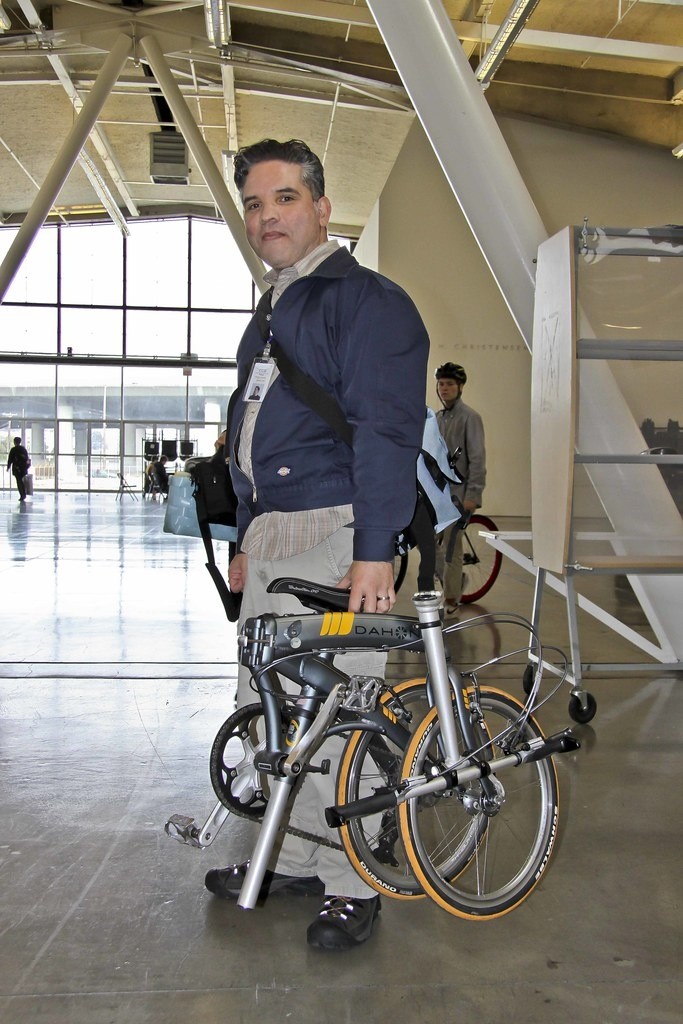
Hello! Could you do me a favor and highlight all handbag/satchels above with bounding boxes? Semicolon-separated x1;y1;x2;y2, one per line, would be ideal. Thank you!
385;399;467;551
164;466;242;546
23;472;34;496
190;450;241;527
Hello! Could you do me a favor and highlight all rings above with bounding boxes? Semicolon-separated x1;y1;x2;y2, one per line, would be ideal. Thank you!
376;595;390;602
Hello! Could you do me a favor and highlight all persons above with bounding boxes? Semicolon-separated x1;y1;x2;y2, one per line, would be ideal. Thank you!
6;436;29;501
433;361;488;621
206;135;435;953
154;455;169;493
146;456;159;481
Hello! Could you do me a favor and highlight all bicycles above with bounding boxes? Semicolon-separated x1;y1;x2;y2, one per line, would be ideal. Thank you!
165;575;577;926
357;446;505;608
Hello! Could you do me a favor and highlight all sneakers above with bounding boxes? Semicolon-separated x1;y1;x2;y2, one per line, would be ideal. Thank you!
435;601;459;623
305;889;382;947
202;859;322;901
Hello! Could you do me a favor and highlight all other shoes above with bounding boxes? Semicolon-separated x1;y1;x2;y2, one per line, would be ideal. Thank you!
18;494;26;500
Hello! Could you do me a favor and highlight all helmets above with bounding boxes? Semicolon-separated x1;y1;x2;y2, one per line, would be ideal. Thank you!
435;363;469;379
